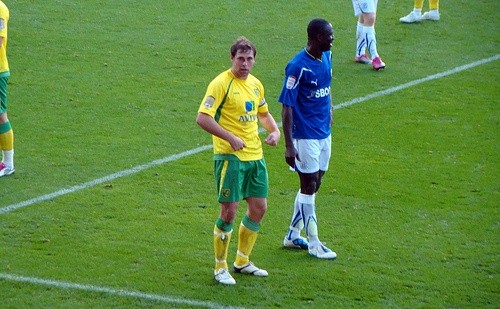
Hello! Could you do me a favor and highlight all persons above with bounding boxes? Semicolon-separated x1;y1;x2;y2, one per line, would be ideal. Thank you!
352;0;386;69
0;0;16;177
399;0;440;23
196;36;280;285
278;19;337;259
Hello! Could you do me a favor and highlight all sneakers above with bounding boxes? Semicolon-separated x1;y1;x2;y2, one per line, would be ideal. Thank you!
354;54;373;64
399;11;422;22
233;261;269;277
283;234;308;248
213;267;236;285
422;11;440;21
307;241;337;259
372;56;385;70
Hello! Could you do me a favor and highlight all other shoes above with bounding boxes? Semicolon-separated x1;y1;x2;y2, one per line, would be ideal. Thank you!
0;162;15;177
